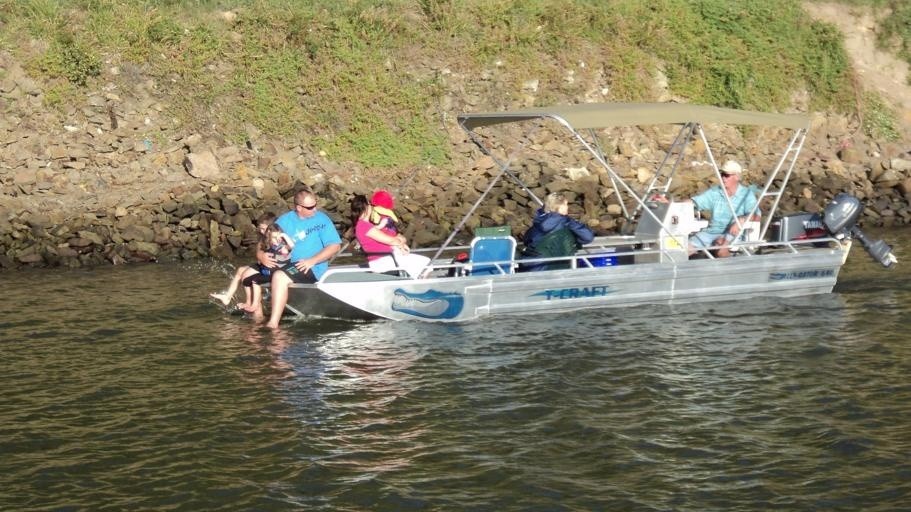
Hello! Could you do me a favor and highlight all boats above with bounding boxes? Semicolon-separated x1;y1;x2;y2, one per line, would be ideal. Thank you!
246;97;899;320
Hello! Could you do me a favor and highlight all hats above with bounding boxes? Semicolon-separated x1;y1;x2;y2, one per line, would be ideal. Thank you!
718;160;742;175
370;191;394;209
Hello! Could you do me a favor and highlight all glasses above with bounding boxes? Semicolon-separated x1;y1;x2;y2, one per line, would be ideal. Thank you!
720;173;736;177
296;201;317;210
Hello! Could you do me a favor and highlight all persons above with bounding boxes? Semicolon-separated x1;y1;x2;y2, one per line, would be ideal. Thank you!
209;212;277;313
370;191;410;256
351;194;434;279
648;159;761;258
236;222;296;312
248;189;343;328
514;192;594;272
